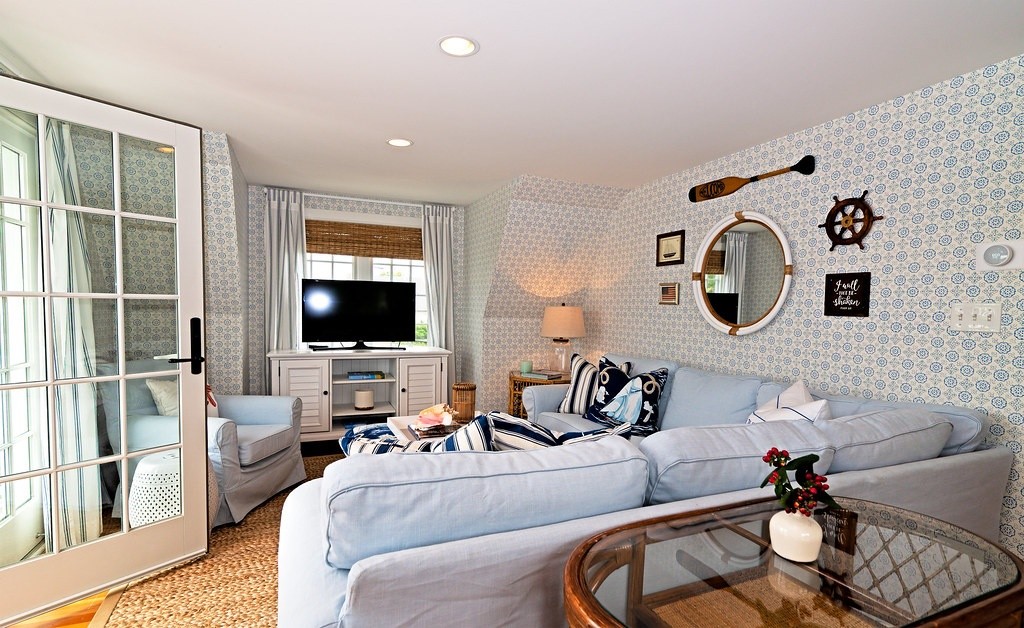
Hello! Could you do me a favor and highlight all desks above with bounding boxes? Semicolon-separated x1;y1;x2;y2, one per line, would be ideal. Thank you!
565;492;1024;628
128;448;218;537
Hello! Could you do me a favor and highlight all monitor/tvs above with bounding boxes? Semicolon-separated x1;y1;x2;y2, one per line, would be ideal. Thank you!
301;278;415;350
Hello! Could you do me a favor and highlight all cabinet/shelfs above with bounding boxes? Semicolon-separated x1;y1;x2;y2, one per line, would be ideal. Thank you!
266;344;449;441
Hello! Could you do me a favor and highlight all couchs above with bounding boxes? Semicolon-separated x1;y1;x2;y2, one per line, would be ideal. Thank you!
278;352;1014;628
95;360;307;530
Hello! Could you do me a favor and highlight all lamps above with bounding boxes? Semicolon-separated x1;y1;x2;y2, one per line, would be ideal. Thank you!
541;303;587;374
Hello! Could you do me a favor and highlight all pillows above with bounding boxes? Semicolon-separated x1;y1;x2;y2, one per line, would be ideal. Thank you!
746;379;832;425
145;378;219;416
338;415;490;457
485;412;631;450
556;353;631;415
583;355;668;437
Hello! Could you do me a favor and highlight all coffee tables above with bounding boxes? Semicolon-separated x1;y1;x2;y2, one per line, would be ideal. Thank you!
387;411;493;441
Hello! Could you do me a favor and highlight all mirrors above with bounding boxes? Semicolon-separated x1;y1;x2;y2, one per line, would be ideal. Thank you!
692;211;793;336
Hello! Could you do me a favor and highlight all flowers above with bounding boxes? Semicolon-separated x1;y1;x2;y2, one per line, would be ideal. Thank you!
761;447;829;518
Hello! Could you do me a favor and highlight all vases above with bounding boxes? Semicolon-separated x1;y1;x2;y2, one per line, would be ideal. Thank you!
769;510;822;562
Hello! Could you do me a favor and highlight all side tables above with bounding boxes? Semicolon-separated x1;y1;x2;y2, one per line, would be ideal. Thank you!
508;370;571;419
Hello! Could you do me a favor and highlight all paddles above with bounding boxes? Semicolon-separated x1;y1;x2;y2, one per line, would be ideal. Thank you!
687;155;816;203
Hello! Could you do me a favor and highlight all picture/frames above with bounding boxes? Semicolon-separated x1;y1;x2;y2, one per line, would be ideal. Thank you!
656;230;685;266
658;283;679;305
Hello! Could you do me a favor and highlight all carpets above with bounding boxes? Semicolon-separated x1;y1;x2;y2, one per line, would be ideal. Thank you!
88;453;345;628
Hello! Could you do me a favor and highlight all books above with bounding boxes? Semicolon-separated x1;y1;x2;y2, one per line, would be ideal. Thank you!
347;370;386;379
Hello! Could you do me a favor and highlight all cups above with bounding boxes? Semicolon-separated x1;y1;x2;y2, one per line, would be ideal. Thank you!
519;360;533;377
